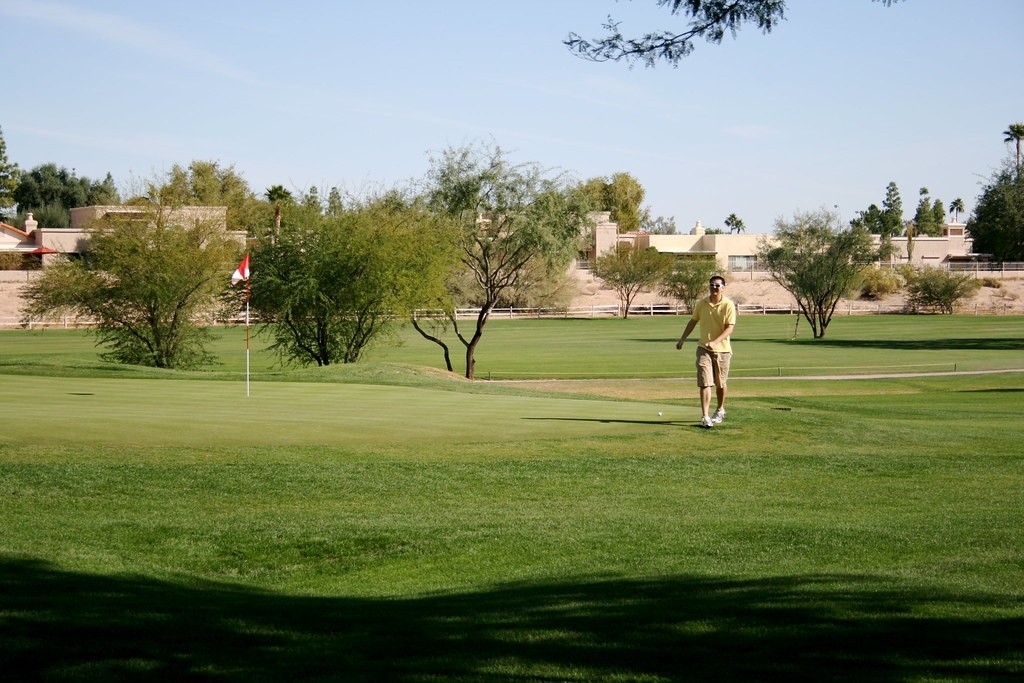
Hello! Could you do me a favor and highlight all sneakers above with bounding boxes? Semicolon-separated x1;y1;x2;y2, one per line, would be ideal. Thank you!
711;409;725;423
703;417;713;428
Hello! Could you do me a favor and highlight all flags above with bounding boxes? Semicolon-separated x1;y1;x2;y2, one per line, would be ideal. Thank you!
231;252;250;288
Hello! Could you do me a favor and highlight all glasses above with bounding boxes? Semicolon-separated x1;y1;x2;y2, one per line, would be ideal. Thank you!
710;283;724;287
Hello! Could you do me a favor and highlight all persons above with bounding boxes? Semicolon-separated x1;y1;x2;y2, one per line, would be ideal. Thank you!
676;276;736;426
